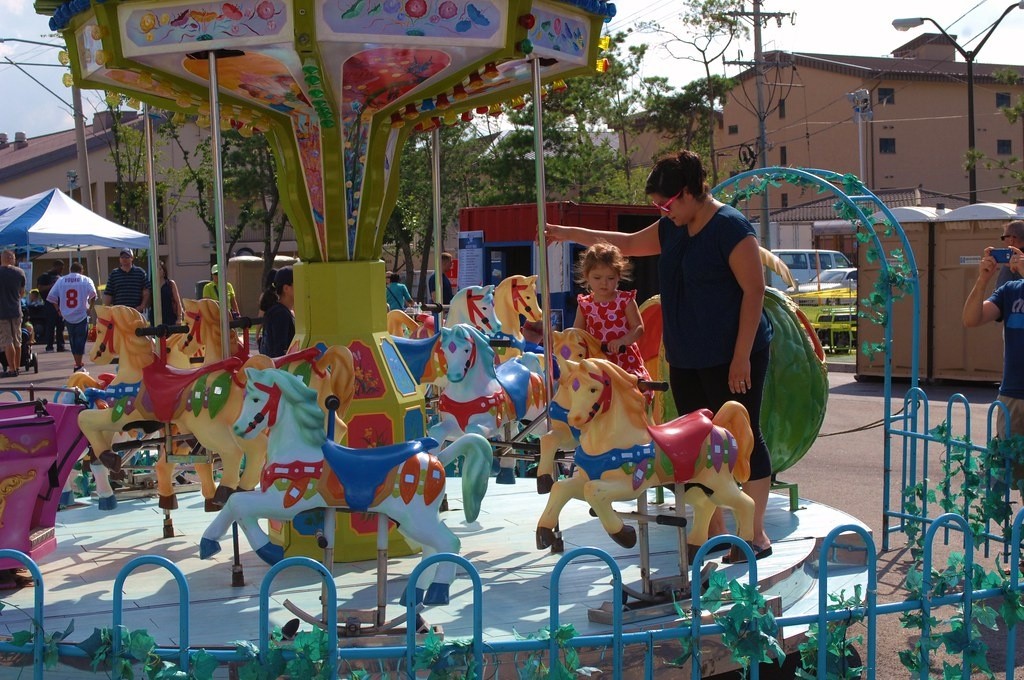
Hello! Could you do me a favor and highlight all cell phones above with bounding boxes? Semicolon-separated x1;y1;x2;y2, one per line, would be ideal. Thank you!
989;248;1013;263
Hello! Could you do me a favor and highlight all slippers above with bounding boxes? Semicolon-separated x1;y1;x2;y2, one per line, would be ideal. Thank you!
723;544;774;563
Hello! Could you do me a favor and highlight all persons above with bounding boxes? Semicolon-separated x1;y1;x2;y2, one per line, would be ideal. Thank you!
571;241;655;403
103;249;149;313
46;262;98;374
385;271;413;311
962;247;1024;492
429;252;453;333
993;220;1024;288
147;261;182;326
257;266;295;356
202;265;242;318
536;149;771;562
29;288;42;306
0;249;25;378
38;261;65;352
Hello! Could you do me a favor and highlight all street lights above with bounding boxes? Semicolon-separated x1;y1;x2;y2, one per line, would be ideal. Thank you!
892;1;1024;207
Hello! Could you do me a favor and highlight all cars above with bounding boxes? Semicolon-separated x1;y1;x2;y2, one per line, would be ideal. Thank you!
786;268;858;309
768;249;855;299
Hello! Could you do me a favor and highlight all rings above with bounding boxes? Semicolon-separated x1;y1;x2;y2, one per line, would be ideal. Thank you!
739;381;745;385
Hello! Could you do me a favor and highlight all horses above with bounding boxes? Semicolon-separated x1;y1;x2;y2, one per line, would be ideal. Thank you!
76;273;760;608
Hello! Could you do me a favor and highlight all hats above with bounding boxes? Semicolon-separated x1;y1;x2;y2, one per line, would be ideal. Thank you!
212;264;218;274
120;249;133;256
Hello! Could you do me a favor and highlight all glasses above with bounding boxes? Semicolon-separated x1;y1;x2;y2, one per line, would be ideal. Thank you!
652;190;687;213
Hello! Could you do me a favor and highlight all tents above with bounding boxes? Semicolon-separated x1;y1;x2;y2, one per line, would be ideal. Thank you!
1;187;150;292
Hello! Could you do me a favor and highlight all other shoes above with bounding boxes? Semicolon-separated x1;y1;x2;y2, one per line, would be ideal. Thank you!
57;348;70;353
45;345;55;353
1;369;19;377
